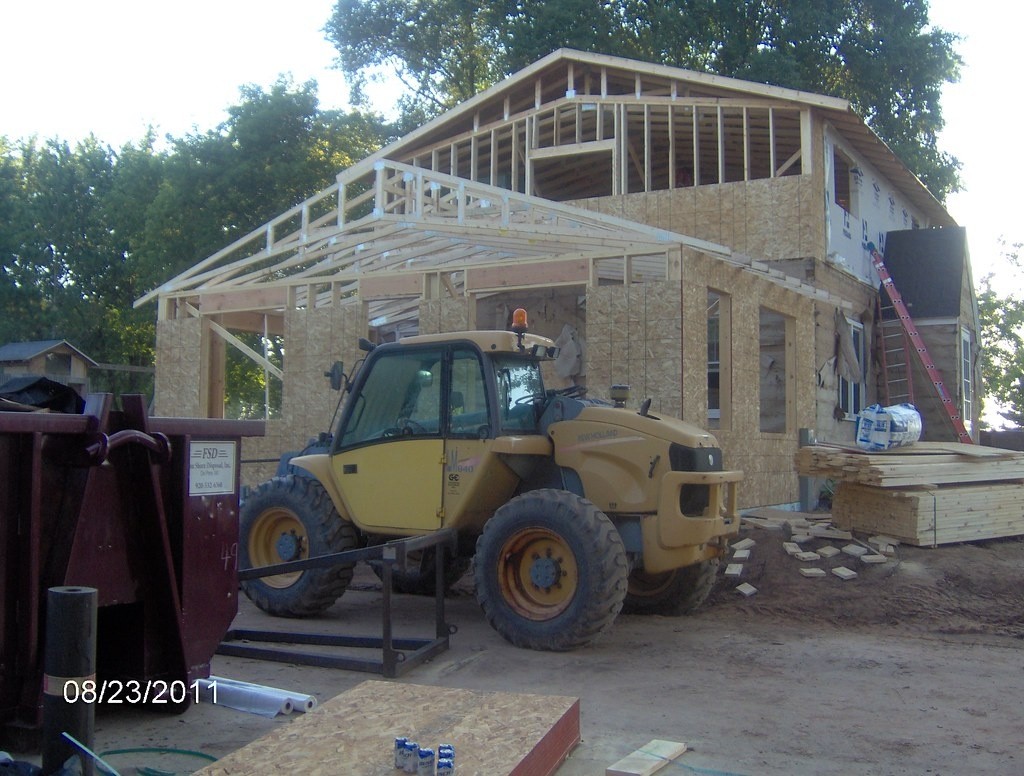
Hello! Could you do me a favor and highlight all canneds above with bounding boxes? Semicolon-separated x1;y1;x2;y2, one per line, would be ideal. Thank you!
438;744;454;760
437;758;454;776
440;750;455;771
418;749;435;776
403;742;419;773
395;738;409;767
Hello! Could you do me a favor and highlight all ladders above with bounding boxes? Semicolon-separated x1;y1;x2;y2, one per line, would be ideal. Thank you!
866;240;977;444
877;290;914;407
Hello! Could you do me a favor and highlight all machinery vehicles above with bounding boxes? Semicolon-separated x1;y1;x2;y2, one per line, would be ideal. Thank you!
238;307;747;652
